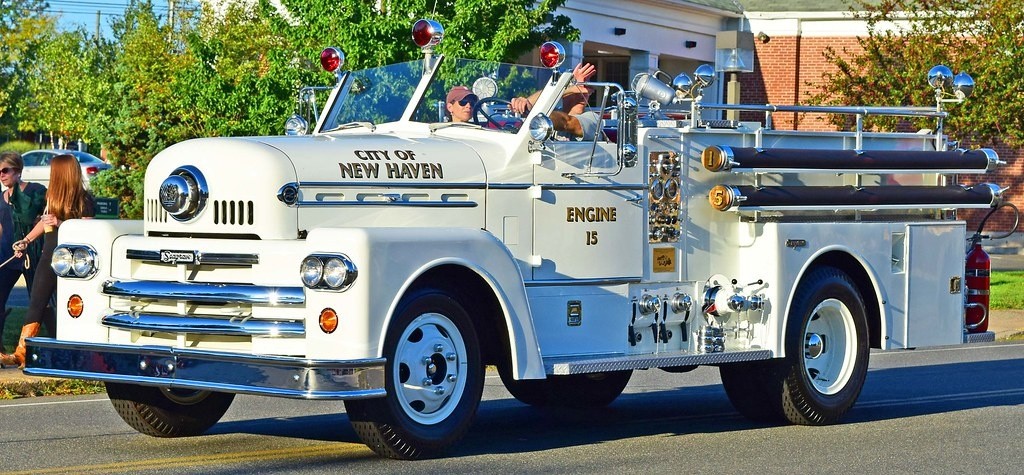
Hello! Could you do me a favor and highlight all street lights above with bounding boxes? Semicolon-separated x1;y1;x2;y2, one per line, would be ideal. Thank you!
714;30;754;121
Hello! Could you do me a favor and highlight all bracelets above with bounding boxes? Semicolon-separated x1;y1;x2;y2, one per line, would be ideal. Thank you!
25;236;30;243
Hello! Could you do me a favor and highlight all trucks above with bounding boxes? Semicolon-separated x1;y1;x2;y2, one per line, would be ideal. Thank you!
21;19;1019;459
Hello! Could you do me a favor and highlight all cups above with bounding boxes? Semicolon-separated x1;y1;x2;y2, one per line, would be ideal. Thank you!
40;214;54;233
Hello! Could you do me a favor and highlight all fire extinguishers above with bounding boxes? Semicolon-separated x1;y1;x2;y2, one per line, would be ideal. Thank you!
965;202;1020;333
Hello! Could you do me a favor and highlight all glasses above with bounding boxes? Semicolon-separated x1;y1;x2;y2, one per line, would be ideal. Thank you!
458;98;475;106
0;166;12;174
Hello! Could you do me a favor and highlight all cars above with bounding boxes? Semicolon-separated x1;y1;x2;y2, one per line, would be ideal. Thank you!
0;149;114;192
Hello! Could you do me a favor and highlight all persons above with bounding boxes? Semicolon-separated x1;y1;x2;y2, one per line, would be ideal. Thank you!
446;86;478;123
0;152;48;312
0;154;96;370
510;62;603;142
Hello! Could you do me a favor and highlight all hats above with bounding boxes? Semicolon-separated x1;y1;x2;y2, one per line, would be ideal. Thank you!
447;86;478;103
562;85;588;98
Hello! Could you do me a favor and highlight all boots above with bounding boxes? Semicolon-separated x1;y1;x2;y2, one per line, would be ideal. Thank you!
0;322;40;370
